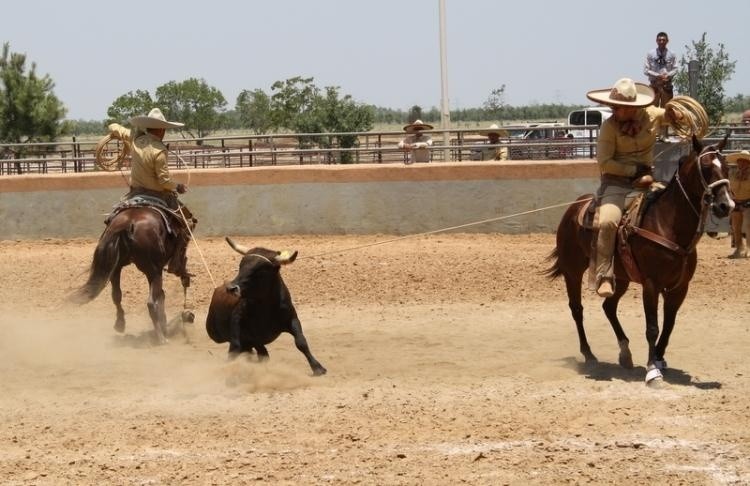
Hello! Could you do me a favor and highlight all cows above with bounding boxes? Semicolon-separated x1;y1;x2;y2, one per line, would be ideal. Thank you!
205;235;328;378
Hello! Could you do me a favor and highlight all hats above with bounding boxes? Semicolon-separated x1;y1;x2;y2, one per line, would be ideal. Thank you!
478;124;506;138
130;106;185;130
586;78;657;108
726;150;750;164
403;119;434;131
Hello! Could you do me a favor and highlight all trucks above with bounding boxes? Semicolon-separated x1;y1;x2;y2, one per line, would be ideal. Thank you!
567;106;615;156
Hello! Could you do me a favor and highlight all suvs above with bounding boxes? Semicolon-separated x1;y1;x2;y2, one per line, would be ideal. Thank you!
470;121;568;159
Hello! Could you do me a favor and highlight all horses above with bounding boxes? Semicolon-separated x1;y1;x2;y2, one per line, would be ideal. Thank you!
535;130;735;391
66;195;197;346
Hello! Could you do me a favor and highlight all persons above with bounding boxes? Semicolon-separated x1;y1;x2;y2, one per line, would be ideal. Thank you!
725;150;750;258
108;108;193;273
587;78;692;297
399;120;433;163
469;124;508;160
643;32;678;135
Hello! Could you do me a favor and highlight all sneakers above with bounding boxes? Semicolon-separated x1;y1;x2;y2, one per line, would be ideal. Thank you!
596;272;616;298
725;247;750;259
167;258;198;278
